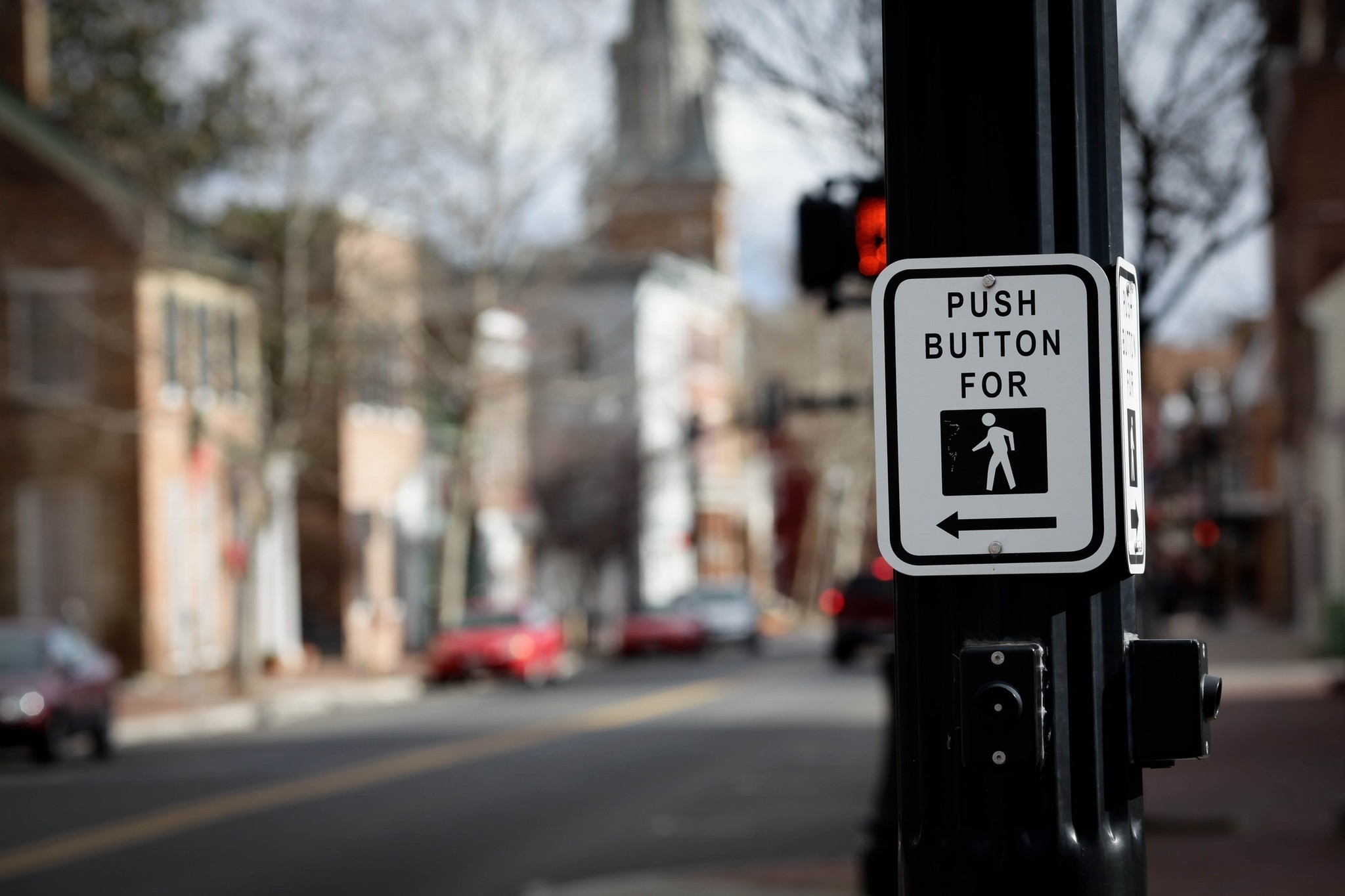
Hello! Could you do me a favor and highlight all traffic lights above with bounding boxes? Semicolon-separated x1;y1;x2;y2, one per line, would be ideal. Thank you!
791;176;885;299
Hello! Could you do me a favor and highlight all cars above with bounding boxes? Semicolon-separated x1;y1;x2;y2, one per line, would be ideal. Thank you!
423;597;567;688
0;611;129;765
823;560;898;664
620;578;761;655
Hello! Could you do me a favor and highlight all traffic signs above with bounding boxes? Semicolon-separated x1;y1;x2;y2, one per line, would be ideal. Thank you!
871;254;1134;578
